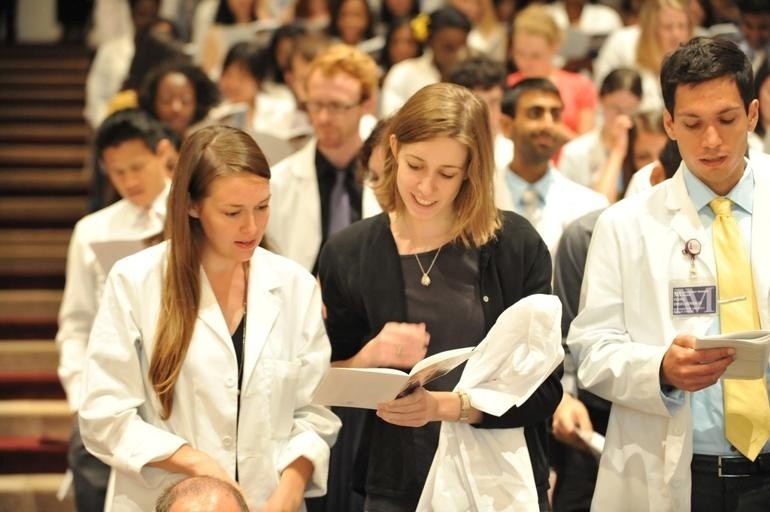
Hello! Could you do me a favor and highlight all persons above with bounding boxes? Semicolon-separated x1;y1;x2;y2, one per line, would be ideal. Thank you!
441;55;515;176
631;0;696;111
754;59;770;146
316;81;563;512
723;0;770;79
330;1;375;47
75;125;342;512
501;7;600;167
203;0;272;80
451;0;509;65
263;44;383;279
85;0;164;131
278;45;378;136
559;66;645;197
106;30;194;116
290;0;330;45
380;0;421;30
359;115;396;213
381;6;473;120
553;137;684;512
593;107;669;204
379;13;427;72
489;78;611;293
268;22;310;80
138;60;218;169
562;6;625;77
218;43;295;146
57;109;185;512
567;35;770;512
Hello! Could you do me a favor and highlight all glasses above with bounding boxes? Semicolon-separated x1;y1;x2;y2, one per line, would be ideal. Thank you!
305;99;362;114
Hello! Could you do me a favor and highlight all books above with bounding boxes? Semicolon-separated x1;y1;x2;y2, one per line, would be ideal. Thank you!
695;329;770;380
254;128;316;167
309;346;476;411
88;228;167;275
184;103;246;137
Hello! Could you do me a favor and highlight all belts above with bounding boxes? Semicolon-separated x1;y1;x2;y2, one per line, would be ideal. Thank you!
688;453;767;478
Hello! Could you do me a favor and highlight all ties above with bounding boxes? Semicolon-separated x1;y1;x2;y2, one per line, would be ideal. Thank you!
329;170;350;239
707;197;770;462
520;189;540;231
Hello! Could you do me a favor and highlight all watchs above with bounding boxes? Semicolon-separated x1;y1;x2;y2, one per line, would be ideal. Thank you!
455;391;471;424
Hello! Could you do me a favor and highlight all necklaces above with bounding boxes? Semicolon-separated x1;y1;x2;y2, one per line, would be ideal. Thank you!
403;210;457;287
